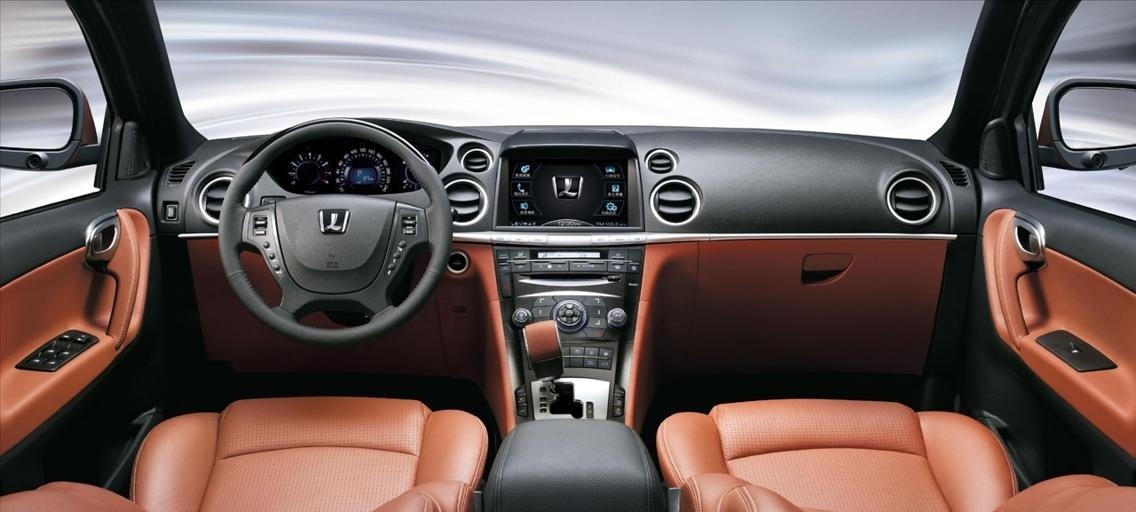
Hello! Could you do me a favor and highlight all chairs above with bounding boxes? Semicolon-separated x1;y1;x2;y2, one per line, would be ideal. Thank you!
650;396;1135;510
2;394;492;510
